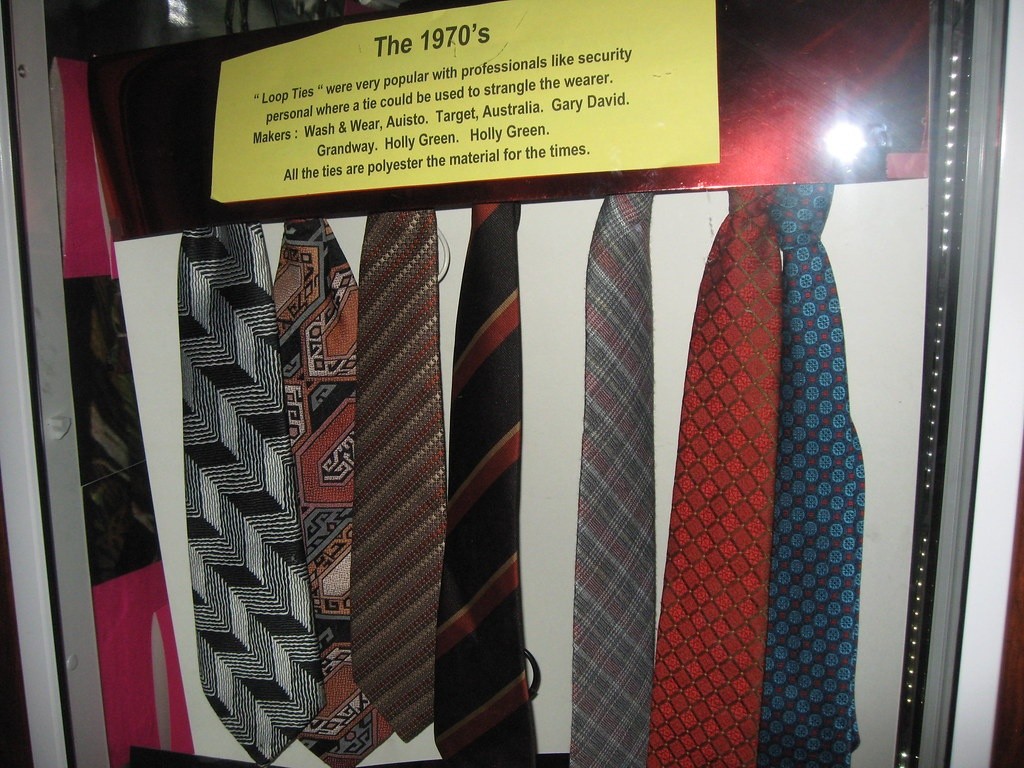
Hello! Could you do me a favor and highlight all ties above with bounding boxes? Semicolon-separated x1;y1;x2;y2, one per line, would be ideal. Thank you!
349;209;440;744
176;221;327;768
757;184;861;768
646;185;782;768
273;219;396;768
569;192;656;768
432;203;536;768
